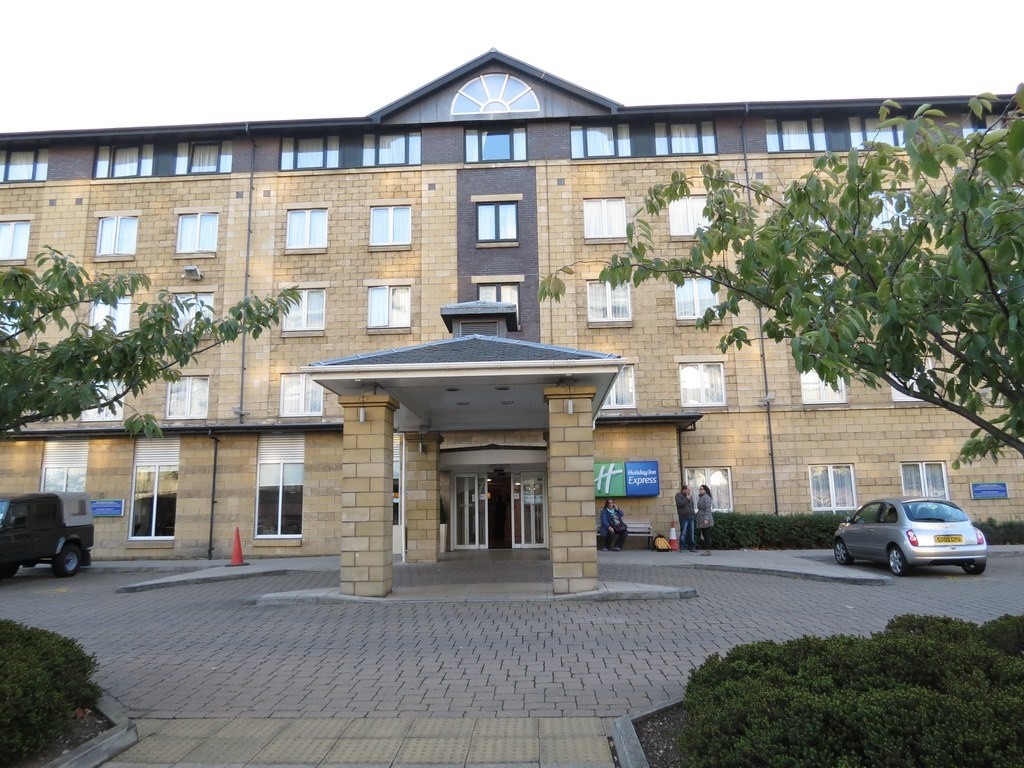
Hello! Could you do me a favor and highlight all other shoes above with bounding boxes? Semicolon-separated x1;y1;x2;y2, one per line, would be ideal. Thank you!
613;547;620;551
700;550;711;556
690;547;697;552
680;549;685;553
602;547;608;551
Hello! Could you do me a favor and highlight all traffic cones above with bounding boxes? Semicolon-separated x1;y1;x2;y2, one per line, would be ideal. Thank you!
667;521;680;552
224;527;250;567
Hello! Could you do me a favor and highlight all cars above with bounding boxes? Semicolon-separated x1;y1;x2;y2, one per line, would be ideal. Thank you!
832;496;988;576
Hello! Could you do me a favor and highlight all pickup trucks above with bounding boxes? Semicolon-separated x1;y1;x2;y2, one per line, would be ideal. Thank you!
0;491;95;579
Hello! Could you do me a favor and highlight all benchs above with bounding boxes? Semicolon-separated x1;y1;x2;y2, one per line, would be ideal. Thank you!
596;516;653;551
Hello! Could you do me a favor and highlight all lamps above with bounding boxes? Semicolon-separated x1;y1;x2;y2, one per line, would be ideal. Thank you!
359;394;364;422
419;427;422;452
231;406;249;416
568;385;573;414
183;266;201;280
758;392;775;409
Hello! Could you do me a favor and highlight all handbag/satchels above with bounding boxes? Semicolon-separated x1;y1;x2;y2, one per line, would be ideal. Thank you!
613;520;627;533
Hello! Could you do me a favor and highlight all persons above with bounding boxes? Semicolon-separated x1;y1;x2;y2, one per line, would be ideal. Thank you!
600;499;628;551
675;485;698;553
695;485;714;556
